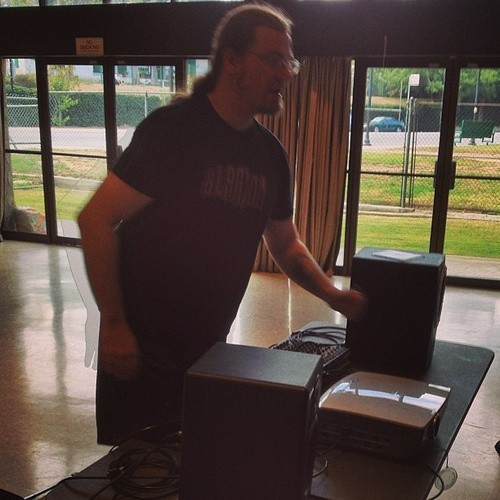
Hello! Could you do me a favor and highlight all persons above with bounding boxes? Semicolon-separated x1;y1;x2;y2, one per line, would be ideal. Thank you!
74;4;367;453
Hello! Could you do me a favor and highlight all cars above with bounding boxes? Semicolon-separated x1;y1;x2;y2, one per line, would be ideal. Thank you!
363;116;406;134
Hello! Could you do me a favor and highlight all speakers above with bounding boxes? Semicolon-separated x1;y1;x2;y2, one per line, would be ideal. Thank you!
177;343;322;499
345;247;447;375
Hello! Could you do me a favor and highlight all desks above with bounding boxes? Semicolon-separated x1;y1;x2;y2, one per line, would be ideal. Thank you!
20;319;494;500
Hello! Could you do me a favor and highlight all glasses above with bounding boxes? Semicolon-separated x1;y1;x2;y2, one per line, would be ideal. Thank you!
238;46;300;75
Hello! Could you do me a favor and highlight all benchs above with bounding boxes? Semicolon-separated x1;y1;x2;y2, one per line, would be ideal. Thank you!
453;118;495;146
